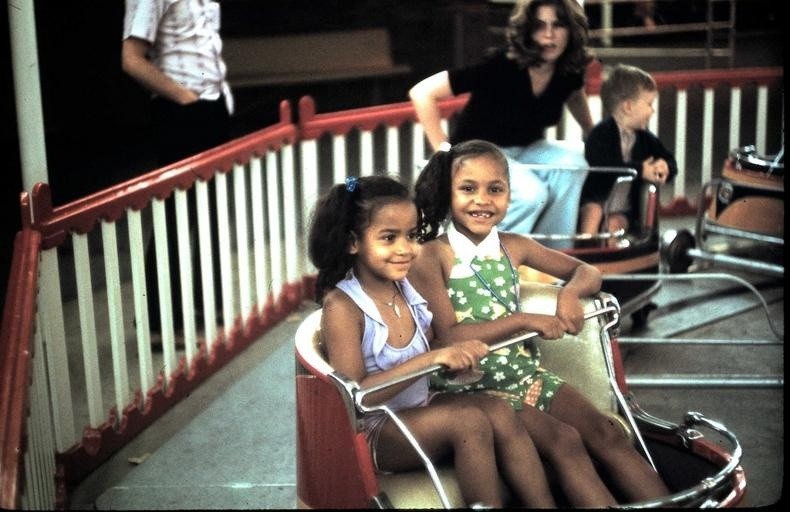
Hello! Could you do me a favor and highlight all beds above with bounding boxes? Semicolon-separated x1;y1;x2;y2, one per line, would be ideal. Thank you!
292;288;637;512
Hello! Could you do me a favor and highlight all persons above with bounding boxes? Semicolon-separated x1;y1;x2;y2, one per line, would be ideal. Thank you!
407;140;680;510
577;63;678;248
120;0;241;352
407;1;596;252
306;175;561;510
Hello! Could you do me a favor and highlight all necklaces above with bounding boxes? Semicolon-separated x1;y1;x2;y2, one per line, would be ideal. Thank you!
361;283;403;320
